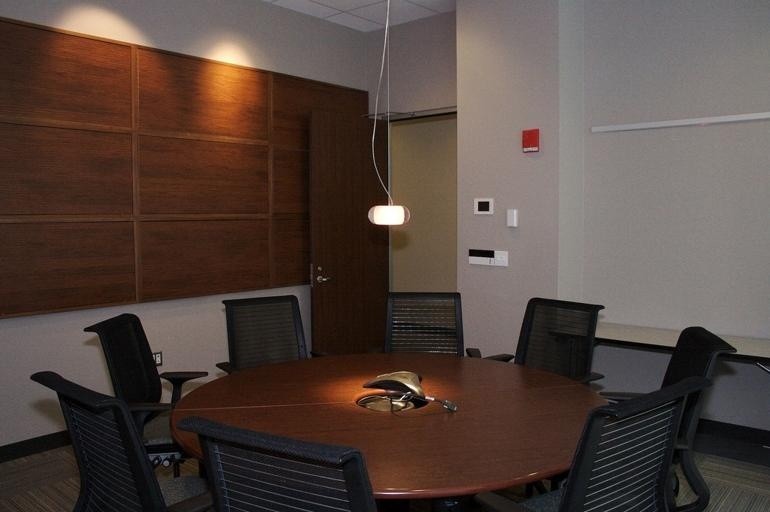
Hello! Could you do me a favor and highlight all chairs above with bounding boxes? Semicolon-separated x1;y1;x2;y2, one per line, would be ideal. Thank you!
476;376;710;512
598;326;737;512
387;289;481;357
175;415;378;511
216;295;329;374
84;313;208;477
29;371;214;511
483;298;604;384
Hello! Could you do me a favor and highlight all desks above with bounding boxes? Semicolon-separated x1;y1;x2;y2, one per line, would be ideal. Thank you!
170;352;608;498
596;319;769;377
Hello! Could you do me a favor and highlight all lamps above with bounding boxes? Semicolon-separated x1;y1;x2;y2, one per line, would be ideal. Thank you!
367;0;411;228
